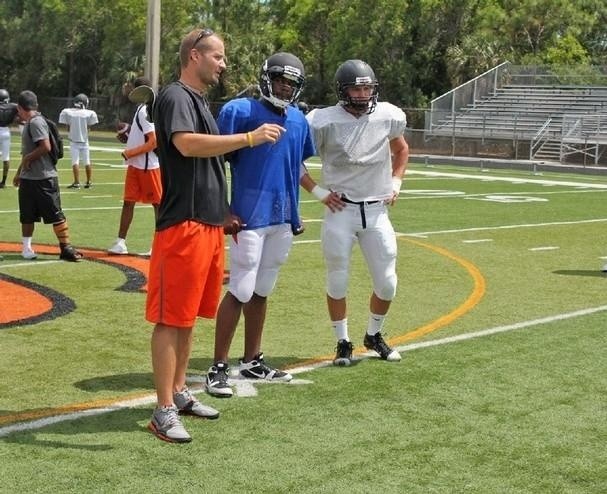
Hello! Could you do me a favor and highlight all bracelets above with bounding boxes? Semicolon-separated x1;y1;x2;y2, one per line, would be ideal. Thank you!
389;174;404;194
246;130;252;148
307;182;332;204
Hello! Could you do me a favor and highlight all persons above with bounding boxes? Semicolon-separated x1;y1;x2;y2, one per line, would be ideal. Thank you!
297;57;411;366
106;76;163;258
12;88;84;262
142;28;287;442
203;52;316;397
0;87;19;189
59;92;99;188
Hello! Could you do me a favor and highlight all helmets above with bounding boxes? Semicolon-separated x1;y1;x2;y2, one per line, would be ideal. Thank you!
0;88;11;103
258;51;306;109
73;92;90;109
334;58;379;116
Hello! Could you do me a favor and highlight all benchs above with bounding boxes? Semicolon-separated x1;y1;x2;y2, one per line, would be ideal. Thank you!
435;82;607;138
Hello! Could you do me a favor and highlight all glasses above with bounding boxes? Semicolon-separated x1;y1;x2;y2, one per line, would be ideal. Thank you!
189;27;214;60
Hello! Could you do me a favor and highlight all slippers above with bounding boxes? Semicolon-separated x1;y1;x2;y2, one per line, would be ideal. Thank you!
60;245;84;259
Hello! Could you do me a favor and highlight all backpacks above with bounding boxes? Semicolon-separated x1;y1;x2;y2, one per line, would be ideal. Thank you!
28;114;64;164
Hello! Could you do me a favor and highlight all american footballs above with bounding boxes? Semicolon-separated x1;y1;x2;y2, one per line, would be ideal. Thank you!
116;122;131;142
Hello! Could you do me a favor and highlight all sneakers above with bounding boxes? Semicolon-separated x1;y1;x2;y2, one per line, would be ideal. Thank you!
173;387;220;419
239;352;293;382
333;338;353;367
67;183;80;190
84;183;94;188
21;249;39;259
364;331;402;362
148;405;192;443
108;245;129;255
205;360;233;397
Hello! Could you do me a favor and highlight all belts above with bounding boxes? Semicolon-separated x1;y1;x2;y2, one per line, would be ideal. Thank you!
340;197;379;229
